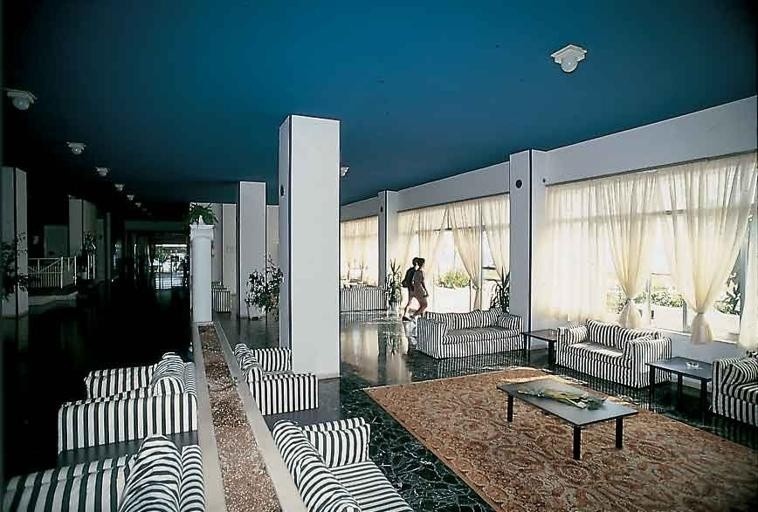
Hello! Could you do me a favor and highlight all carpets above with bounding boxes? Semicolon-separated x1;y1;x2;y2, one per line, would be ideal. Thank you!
360;368;757;512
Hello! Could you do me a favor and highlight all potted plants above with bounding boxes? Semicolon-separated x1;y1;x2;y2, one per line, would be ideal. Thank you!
382;258;403;318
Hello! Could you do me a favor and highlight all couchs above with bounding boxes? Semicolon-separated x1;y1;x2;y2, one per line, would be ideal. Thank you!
272;416;415;512
710;352;756;427
211;281;231;314
55;350;197;454
1;434;206;512
339;286;386;313
234;343;319;417
555;318;670;388
415;308;522;364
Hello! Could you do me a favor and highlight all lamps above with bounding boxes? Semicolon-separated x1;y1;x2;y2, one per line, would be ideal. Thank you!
3;83;155;218
550;43;590;75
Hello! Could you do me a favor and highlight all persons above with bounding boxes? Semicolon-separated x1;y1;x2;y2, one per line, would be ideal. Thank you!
411;257;429;317
400;258;420;322
